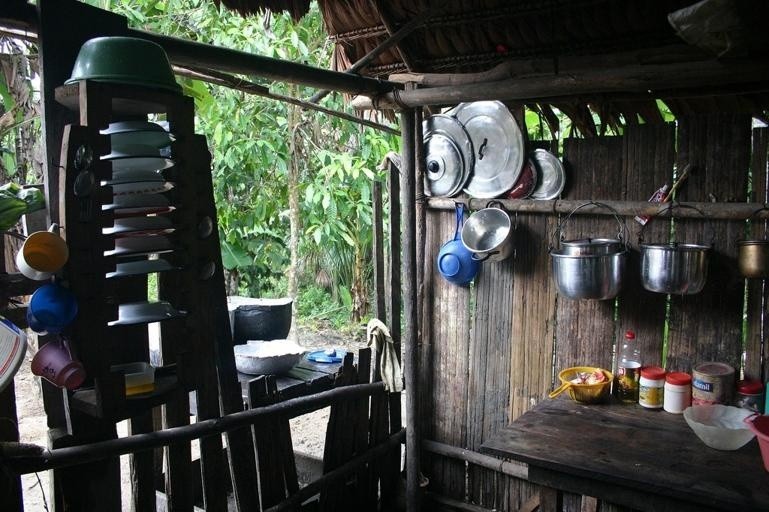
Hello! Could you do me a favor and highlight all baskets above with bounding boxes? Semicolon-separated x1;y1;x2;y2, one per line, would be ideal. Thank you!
548;364;613;404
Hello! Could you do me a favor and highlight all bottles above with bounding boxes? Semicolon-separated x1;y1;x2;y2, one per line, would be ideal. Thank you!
615;331;641;404
634;367;690;416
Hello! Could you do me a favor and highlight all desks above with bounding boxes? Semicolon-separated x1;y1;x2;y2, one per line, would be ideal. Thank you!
231;350;355;402
477;388;769;511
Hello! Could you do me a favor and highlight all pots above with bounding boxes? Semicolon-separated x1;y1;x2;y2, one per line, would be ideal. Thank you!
460;200;518;270
736;240;769;280
550;235;628;301
639;241;712;295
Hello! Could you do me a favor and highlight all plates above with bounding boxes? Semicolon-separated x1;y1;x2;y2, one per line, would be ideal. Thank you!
95;122;191;327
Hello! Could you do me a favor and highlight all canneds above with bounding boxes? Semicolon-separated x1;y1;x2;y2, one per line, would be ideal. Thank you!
692;361;735;406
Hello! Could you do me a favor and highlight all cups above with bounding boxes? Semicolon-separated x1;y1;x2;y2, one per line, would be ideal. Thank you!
31;339;89;391
25;280;85;340
16;221;69;282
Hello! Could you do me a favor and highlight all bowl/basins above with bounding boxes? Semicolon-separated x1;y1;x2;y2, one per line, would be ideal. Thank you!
63;35;184;92
683;403;760;450
